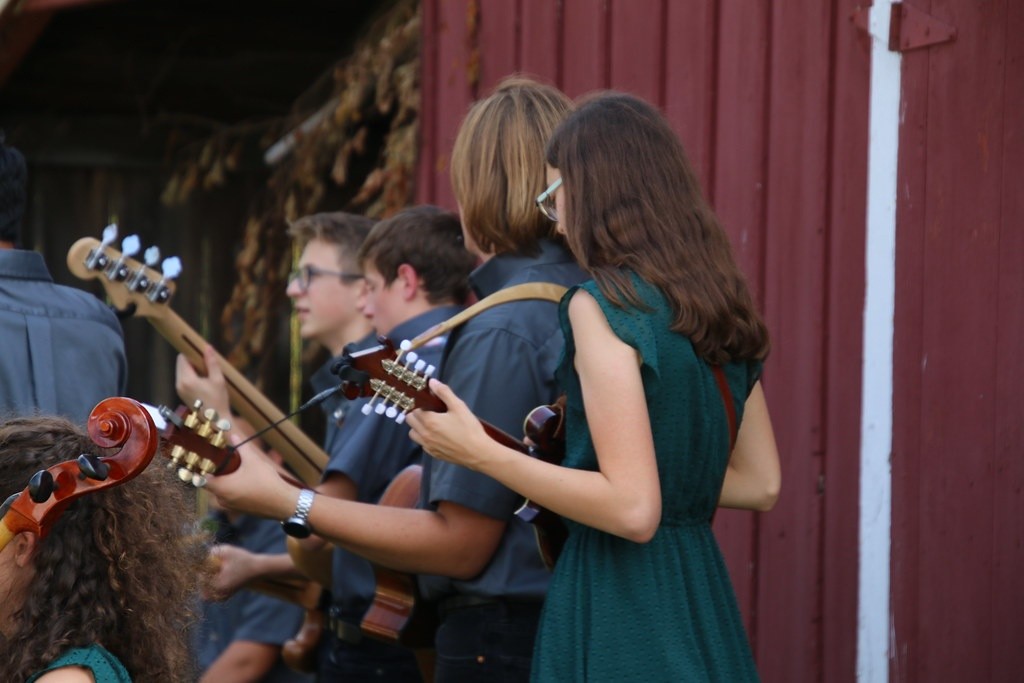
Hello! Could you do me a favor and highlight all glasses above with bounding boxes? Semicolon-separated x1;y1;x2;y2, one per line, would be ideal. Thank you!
536;176;563;221
287;265;364;291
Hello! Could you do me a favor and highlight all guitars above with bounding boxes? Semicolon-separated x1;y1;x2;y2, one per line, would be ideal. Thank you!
155;404;441;683
63;223;391;571
326;330;573;570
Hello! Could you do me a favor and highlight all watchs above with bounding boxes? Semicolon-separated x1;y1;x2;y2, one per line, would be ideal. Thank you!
279;489;317;539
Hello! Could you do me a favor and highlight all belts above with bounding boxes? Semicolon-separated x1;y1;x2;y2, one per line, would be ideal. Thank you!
449;601;542;620
319;603;369;644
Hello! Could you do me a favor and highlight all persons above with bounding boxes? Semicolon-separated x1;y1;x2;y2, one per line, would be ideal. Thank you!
1;145;129;426
0;416;208;683
201;80;784;683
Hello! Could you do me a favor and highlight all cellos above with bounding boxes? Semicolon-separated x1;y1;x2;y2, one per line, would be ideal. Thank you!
0;397;157;558
